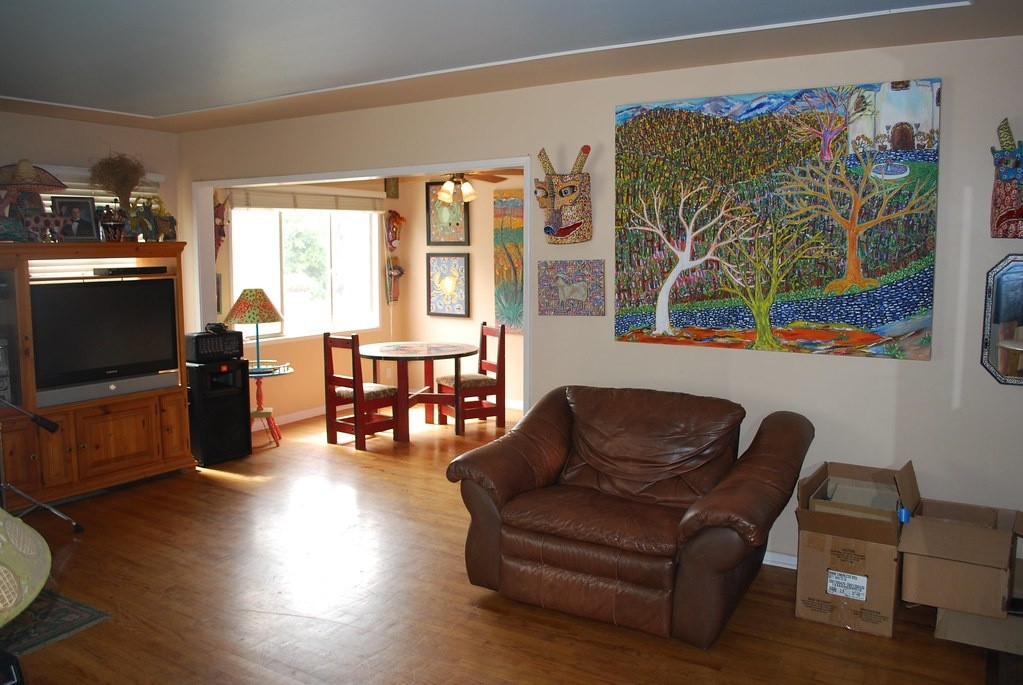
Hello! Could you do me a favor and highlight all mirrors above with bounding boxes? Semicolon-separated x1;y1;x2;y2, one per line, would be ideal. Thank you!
980;254;1023;386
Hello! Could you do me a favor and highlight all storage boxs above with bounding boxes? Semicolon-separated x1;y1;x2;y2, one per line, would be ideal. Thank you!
795;461;1023;657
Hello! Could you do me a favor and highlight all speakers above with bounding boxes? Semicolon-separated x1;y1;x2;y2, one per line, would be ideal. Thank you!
183;359;251;466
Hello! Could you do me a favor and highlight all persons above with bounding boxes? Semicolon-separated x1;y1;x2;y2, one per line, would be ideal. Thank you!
63;207;94;236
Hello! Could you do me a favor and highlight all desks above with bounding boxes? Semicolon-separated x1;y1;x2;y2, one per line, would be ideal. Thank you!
357;341;479;442
249;365;294;447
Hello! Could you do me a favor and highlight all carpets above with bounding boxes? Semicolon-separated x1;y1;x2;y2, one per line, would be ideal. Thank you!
0;588;111;658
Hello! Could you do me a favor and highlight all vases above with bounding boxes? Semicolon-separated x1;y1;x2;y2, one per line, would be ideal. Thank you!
101;222;125;241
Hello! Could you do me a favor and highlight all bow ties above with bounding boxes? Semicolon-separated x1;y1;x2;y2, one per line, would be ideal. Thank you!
71;220;80;223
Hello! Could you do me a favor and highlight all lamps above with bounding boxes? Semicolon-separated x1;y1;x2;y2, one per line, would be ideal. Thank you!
225;288;285;373
437;173;476;203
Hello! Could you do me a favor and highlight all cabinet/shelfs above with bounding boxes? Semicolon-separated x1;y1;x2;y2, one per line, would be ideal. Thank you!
0;240;198;514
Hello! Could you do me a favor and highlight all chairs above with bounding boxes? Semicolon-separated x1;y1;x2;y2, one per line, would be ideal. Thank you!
436;322;505;436
324;332;399;452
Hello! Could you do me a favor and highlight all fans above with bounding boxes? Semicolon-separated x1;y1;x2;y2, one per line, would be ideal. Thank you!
461;168;523;183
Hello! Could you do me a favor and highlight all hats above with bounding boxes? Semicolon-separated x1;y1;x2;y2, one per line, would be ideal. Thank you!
0;158;69;194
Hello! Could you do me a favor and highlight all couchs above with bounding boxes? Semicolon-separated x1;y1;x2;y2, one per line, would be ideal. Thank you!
446;385;815;649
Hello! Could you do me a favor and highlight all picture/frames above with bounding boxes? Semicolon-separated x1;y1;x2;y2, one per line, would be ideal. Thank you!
426;253;470;317
425;182;470;246
51;196;100;242
216;274;222;315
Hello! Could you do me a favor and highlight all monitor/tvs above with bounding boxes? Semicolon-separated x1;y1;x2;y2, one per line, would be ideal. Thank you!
30;276;179;408
